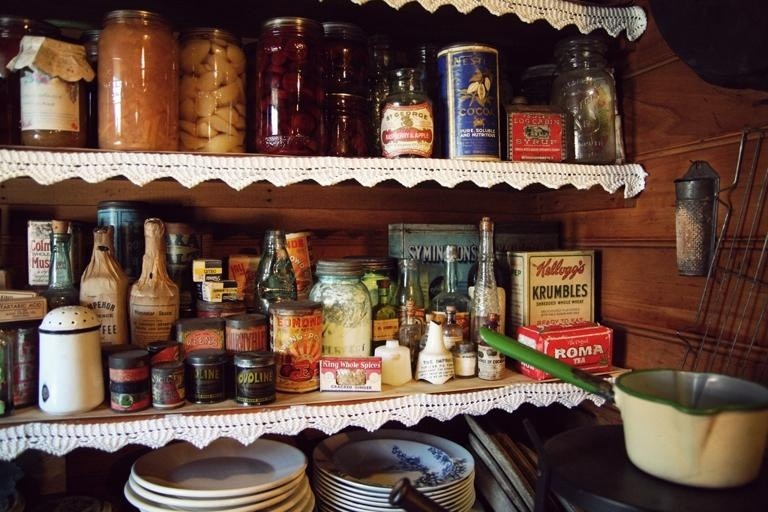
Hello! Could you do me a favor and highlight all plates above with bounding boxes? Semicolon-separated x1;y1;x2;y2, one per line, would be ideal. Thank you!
310;429;475;512
124;438;314;512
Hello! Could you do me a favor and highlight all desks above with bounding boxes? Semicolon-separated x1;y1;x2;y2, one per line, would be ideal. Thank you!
532;423;767;512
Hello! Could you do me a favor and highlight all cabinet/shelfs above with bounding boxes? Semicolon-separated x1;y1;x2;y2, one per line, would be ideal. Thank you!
0;0;647;511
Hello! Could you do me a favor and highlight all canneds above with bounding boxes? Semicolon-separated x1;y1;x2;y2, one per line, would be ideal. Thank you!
96;201;396;414
0;8;369;157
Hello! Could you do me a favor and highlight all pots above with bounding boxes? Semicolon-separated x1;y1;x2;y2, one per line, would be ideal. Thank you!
477;326;766;489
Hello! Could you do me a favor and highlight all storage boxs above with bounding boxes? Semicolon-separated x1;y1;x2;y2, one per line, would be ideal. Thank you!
384;220;565;297
511;319;614;381
496;250;599;340
501;105;576;164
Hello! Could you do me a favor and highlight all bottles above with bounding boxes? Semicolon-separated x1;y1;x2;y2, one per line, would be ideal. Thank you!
255;227;504;384
0;199;178;419
360;29;616;164
470;217;500;353
